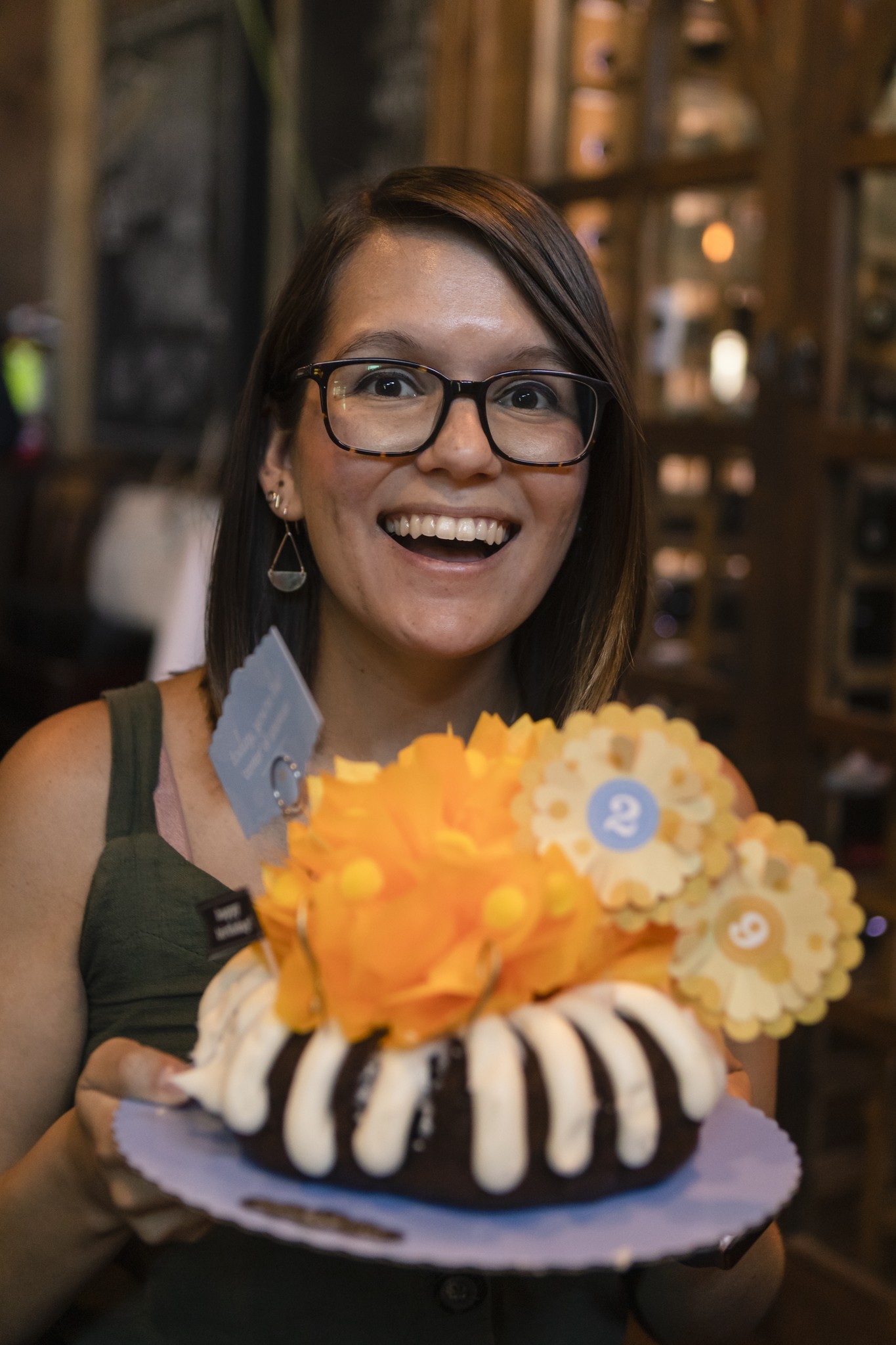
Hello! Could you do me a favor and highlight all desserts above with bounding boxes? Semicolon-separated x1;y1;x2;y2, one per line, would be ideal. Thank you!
163;700;864;1216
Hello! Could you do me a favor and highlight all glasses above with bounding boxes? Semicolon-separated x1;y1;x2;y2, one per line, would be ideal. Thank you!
292;356;610;469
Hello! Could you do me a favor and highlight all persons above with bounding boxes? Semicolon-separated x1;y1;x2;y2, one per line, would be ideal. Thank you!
0;163;789;1345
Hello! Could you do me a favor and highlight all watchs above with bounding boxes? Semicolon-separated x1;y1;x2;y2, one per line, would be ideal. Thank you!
673;1212;773;1272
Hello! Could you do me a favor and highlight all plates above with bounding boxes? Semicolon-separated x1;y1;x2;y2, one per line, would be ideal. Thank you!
109;1085;802;1272
